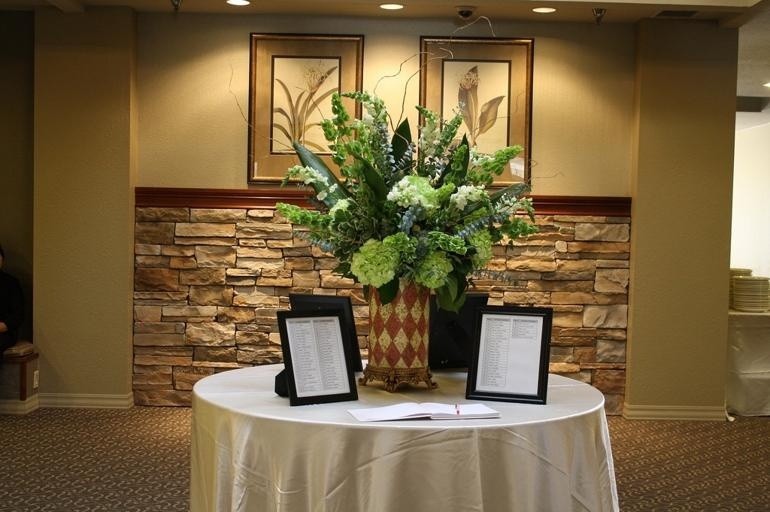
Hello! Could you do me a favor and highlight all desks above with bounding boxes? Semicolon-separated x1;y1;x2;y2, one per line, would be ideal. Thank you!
194;363;607;512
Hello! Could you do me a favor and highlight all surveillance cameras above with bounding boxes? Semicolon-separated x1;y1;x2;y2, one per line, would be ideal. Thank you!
455;6;475;20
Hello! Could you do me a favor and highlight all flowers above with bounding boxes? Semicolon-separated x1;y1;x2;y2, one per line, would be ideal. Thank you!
272;88;541;318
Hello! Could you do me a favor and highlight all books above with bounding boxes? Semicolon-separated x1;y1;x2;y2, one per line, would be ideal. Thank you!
349;403;501;422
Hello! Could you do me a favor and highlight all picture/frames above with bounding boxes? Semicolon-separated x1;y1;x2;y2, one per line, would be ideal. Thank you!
417;35;535;193
246;31;364;190
433;292;554;403
277;294;359;405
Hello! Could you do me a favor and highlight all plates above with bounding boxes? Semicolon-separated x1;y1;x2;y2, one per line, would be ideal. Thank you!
730;268;770;313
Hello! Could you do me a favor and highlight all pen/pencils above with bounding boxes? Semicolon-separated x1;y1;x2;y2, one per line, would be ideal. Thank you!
455;403;460;415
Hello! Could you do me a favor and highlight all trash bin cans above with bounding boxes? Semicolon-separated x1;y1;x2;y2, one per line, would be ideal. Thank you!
0;339;40;415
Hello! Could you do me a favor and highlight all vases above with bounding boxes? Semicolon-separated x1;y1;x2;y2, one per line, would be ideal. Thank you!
358;278;438;394
